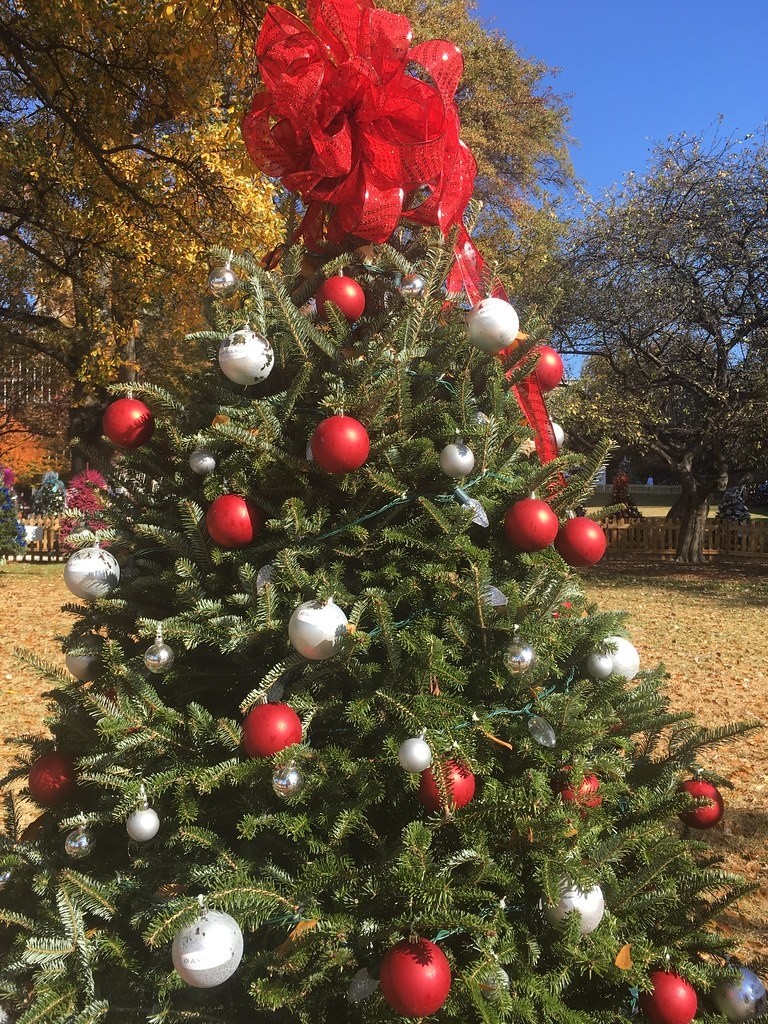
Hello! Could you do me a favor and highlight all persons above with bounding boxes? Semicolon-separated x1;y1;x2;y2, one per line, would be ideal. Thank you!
647;475;654;485
